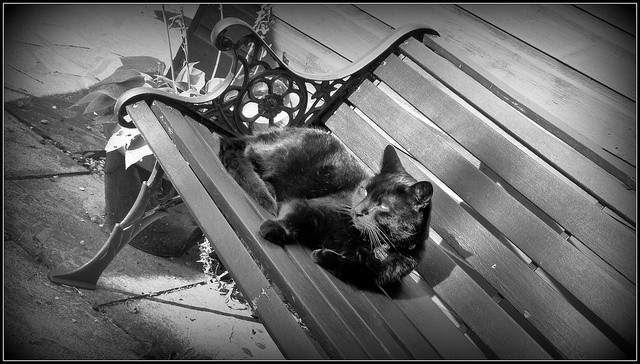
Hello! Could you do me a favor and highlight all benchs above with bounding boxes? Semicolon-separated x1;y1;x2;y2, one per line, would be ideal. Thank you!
47;16;636;361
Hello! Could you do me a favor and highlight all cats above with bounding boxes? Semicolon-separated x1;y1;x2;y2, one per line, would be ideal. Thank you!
219;127;433;298
